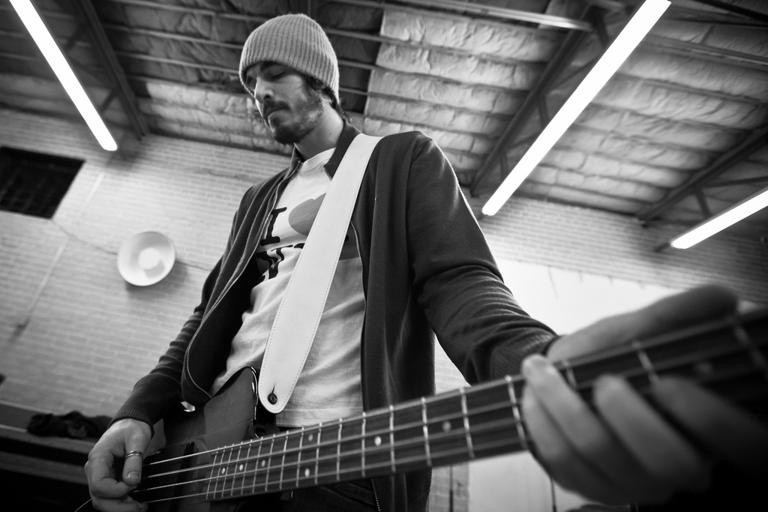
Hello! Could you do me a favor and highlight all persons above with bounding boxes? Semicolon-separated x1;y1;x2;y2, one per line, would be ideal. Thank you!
83;13;767;512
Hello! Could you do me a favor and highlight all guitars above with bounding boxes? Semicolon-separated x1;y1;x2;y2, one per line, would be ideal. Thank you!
77;315;768;512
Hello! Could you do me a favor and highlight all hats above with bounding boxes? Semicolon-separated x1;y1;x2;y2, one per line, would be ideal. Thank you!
234;10;341;110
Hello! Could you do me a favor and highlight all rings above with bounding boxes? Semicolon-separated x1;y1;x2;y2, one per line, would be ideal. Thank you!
124;449;144;458
